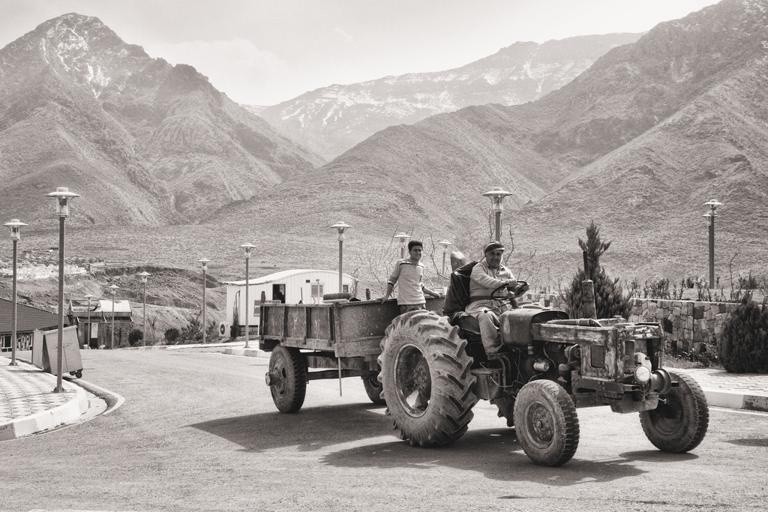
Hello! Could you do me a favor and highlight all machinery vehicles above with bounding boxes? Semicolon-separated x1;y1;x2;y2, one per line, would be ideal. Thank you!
259;251;709;468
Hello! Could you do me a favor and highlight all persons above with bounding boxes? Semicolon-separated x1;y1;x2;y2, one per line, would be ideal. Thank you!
466;239;531;369
374;240;445;314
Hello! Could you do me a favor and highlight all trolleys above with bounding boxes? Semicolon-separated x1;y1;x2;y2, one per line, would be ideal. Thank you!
32;323;83;378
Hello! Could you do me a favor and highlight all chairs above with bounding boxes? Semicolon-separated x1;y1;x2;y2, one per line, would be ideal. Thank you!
442;260;480;338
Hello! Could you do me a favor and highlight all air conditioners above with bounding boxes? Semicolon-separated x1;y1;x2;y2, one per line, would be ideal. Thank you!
218;321;231;338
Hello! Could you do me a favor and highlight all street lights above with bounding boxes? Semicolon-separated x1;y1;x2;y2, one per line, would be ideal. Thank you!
438;239;452;275
330;221;352;293
394;232;412;259
701;199;722;289
482;187;513;242
198;258;211;344
43;187;80;393
84;272;151;349
2;219;29;366
240;242;256;348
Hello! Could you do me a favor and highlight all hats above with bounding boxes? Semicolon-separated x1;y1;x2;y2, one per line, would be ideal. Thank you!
482;241;506;256
